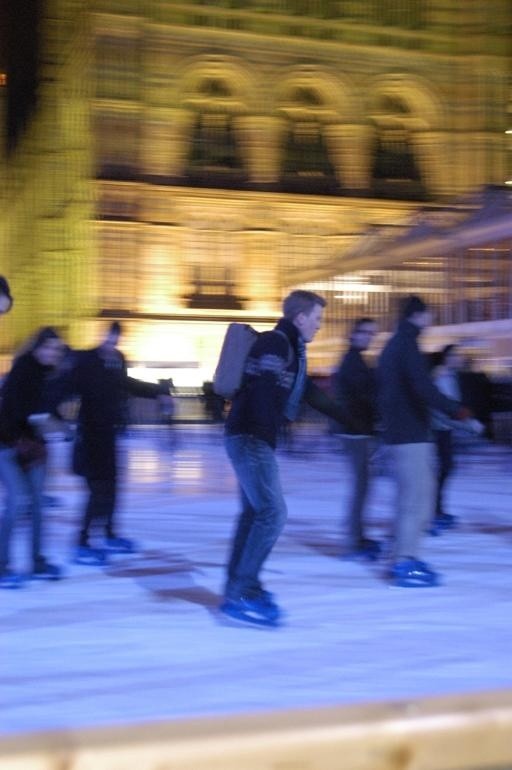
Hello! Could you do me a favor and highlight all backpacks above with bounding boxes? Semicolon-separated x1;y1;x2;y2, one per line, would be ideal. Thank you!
212;323;295;401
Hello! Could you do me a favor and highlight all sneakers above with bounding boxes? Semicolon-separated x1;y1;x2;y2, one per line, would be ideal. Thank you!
76;546;106;561
104;536;133;550
224;582;271;602
224;599;278;620
32;563;58;575
358;512;456;581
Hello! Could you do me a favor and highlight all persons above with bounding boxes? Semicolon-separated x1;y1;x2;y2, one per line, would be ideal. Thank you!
60;321;166;565
1;276;12;314
221;290;341;627
1;327;79;589
326;294;484;588
